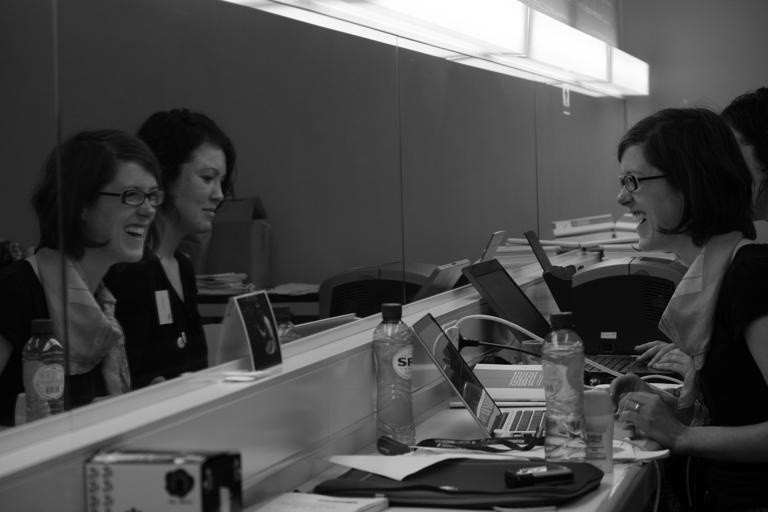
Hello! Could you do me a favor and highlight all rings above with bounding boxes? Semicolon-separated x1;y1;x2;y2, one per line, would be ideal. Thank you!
634;402;640;411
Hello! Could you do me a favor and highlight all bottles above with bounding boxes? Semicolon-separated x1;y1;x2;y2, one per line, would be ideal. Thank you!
541;309;590;461
21;316;67;420
373;303;418;443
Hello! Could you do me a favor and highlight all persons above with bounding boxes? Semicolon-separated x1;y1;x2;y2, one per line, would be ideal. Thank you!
611;109;768;511
103;109;235;390
634;87;768;381
0;130;164;428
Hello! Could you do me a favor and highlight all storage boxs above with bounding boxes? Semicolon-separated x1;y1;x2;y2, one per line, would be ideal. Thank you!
198;196;271;286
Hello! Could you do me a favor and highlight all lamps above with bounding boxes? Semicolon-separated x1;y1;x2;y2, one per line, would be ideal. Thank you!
290;1;650;99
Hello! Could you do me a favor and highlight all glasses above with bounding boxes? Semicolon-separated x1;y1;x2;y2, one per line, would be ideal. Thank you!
95;186;162;207
616;174;666;193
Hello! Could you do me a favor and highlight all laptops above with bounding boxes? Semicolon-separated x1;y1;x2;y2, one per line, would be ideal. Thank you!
413;312;548;441
462;257;682;382
337;462;605;504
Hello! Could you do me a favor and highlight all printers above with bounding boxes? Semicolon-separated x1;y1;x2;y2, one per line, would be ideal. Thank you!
520;230;690;345
316;230;506;324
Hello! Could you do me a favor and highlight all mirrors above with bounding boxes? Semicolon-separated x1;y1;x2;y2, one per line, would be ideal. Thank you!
1;1;627;454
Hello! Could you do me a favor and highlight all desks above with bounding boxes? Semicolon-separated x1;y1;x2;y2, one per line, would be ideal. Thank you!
246;393;662;512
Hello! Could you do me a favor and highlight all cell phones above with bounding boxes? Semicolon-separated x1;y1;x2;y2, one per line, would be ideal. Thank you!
504;463;576;488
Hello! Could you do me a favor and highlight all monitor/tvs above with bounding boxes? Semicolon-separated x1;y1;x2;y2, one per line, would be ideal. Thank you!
291;314;356;338
412;259;471;303
